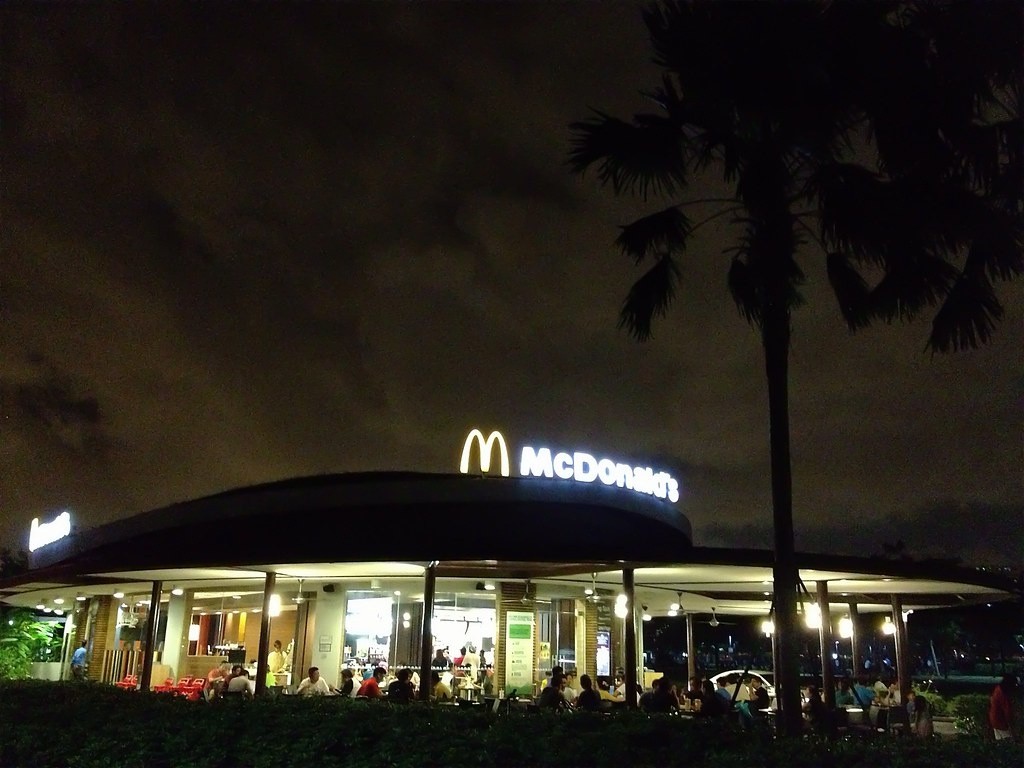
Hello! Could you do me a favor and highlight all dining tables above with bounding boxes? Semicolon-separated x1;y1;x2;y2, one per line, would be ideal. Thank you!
484;697;914;735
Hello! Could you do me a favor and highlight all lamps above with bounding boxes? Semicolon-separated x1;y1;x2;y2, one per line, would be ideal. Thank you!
676;591;685;616
291;578;306;604
520;579;535;604
709;607;720;626
586;572;602;603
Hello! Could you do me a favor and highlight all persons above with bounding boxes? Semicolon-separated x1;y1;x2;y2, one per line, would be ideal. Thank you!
70;640;88;681
537;666;1024;746
205;641;494;704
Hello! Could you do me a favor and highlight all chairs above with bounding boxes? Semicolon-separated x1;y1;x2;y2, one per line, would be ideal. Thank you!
114;674;205;700
